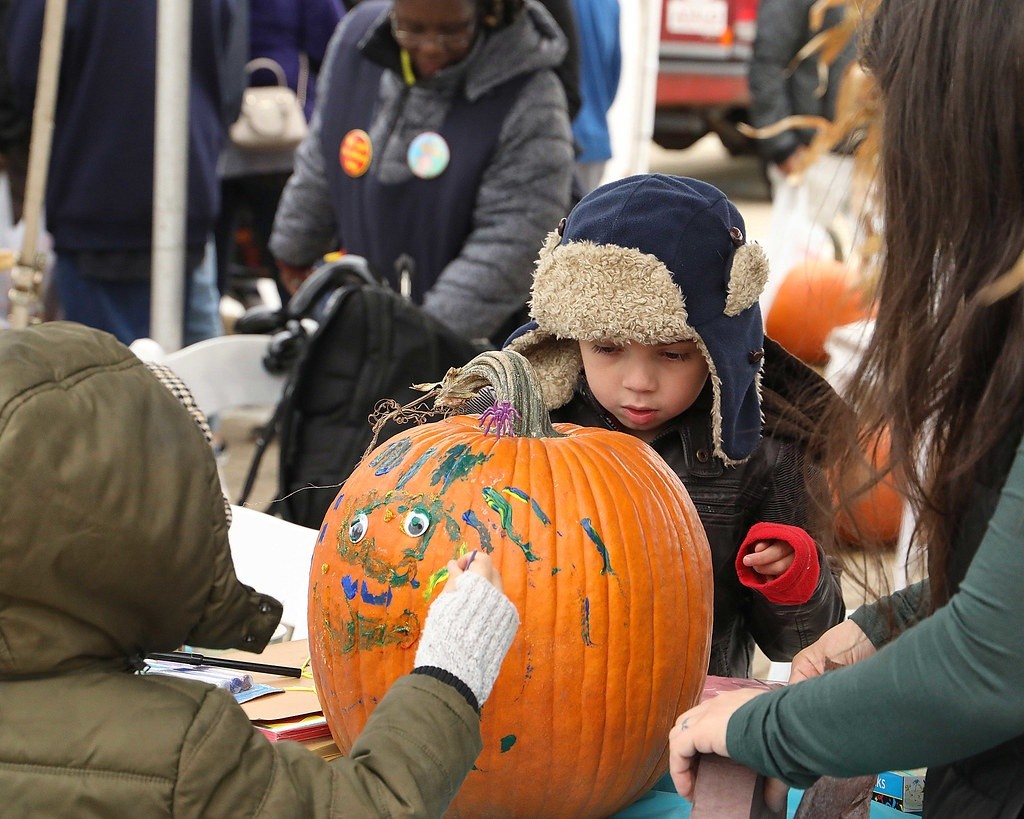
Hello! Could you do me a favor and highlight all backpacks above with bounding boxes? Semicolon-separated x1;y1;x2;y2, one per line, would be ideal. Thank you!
275;285;478;532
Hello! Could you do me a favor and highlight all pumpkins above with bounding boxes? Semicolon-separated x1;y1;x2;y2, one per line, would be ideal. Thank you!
825;421;919;550
767;227;877;368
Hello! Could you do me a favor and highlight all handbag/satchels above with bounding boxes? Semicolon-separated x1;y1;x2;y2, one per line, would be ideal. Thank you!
215;58;310;179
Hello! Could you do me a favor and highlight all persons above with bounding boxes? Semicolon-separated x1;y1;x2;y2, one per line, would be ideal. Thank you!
669;0;1024;819
747;0;880;204
0;0;629;351
0;321;518;819
454;176;859;679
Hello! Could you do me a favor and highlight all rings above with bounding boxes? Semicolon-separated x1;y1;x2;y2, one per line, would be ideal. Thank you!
682;719;688;731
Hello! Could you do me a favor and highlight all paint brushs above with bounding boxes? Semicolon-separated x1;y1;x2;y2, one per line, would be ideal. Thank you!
465;549;478;571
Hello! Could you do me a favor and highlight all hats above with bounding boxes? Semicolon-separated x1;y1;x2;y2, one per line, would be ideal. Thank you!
502;173;767;464
